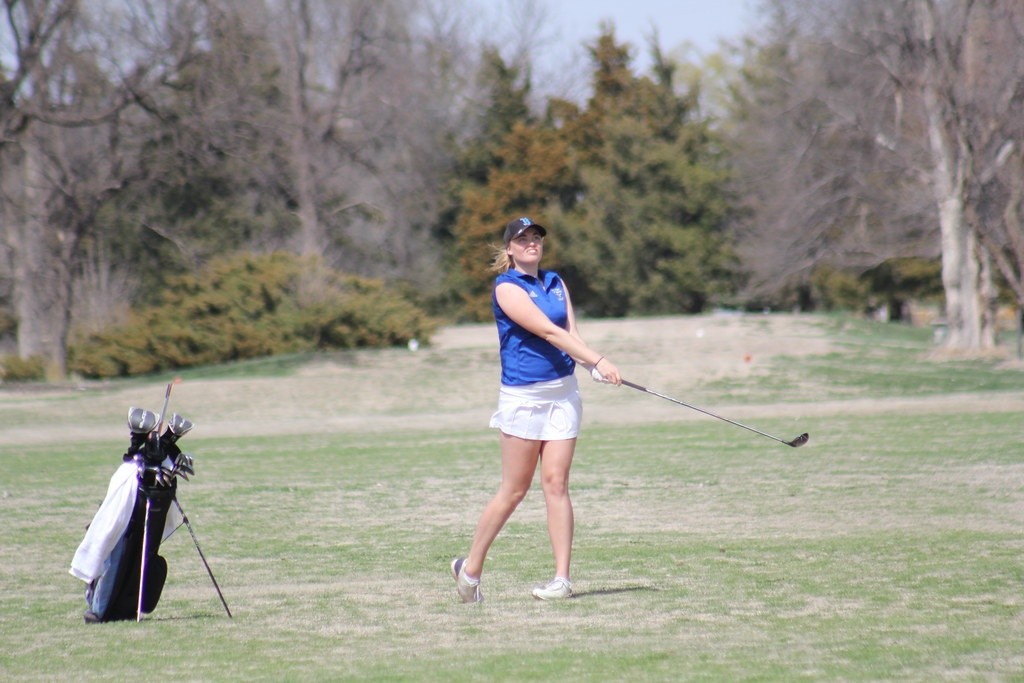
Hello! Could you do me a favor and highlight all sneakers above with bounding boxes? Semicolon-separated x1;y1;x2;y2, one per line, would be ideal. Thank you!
530;577;574;601
450;557;484;603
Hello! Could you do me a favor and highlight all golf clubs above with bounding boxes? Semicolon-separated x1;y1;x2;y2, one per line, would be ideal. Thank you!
130;384;196;486
620;378;809;447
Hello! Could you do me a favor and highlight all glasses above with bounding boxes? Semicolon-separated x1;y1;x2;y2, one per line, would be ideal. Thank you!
510;234;544;244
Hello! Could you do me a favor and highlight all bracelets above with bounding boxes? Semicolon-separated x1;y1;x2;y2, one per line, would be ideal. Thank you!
593;356;604;368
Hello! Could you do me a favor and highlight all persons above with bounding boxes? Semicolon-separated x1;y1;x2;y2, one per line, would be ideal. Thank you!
448;215;621;605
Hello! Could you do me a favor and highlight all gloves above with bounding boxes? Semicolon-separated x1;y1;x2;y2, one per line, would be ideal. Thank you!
586;364;610;384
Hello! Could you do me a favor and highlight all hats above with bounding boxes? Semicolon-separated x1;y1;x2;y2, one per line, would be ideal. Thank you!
503;217;547;248
